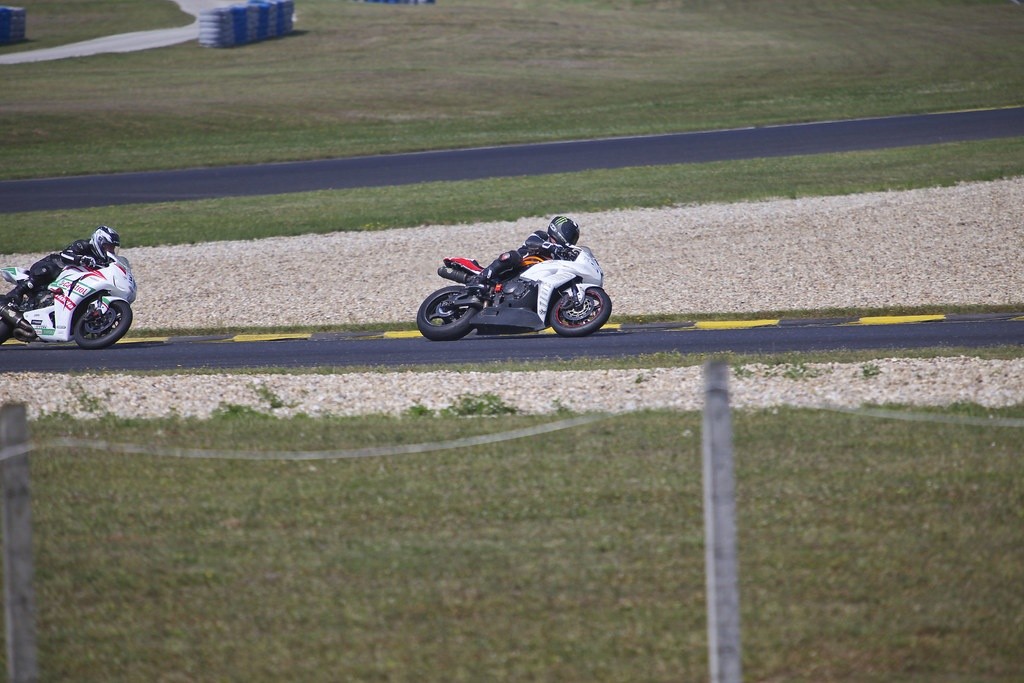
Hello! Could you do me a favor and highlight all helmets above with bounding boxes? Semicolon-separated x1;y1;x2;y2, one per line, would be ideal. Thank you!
548;216;580;246
89;226;121;260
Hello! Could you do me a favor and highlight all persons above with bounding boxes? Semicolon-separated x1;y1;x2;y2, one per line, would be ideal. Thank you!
464;215;581;291
3;224;122;312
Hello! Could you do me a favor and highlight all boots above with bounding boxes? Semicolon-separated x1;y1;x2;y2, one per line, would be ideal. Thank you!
466;260;512;302
4;280;40;313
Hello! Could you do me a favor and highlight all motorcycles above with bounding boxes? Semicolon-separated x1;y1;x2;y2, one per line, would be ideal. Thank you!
0;252;137;350
416;244;613;342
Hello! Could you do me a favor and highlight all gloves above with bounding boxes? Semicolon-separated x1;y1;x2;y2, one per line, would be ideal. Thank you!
81;255;96;269
549;244;564;259
